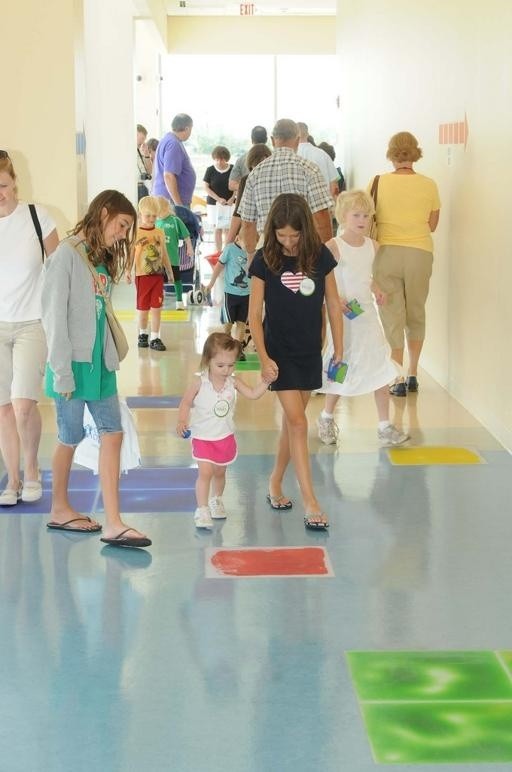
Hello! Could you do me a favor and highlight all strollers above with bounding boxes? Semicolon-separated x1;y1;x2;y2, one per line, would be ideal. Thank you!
156;206;208;305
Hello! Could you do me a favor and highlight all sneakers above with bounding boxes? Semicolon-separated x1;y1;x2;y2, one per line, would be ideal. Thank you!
193;506;214;530
208;494;227;520
376;425;410;445
316;410;339;444
175;300;185;310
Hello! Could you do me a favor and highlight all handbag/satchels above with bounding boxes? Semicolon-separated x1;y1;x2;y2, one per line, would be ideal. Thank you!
73;396;142;478
216;201;235;229
65;236;130;360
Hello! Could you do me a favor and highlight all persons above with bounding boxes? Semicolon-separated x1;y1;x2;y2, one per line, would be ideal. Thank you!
369;131;441;398
176;333;274;528
0;151;60;507
246;194;345;530
124;113;344;360
315;191;409;447
41;190;151;547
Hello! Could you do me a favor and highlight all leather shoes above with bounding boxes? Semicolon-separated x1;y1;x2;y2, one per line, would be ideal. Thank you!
137;333;148;347
0;487;20;506
388;383;407;398
406;375;419;394
20;470;45;504
149;337;166;351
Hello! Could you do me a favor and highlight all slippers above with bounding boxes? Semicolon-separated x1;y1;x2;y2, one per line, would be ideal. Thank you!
45;513;103;533
303;508;329;530
100;526;153;548
265;491;293;510
